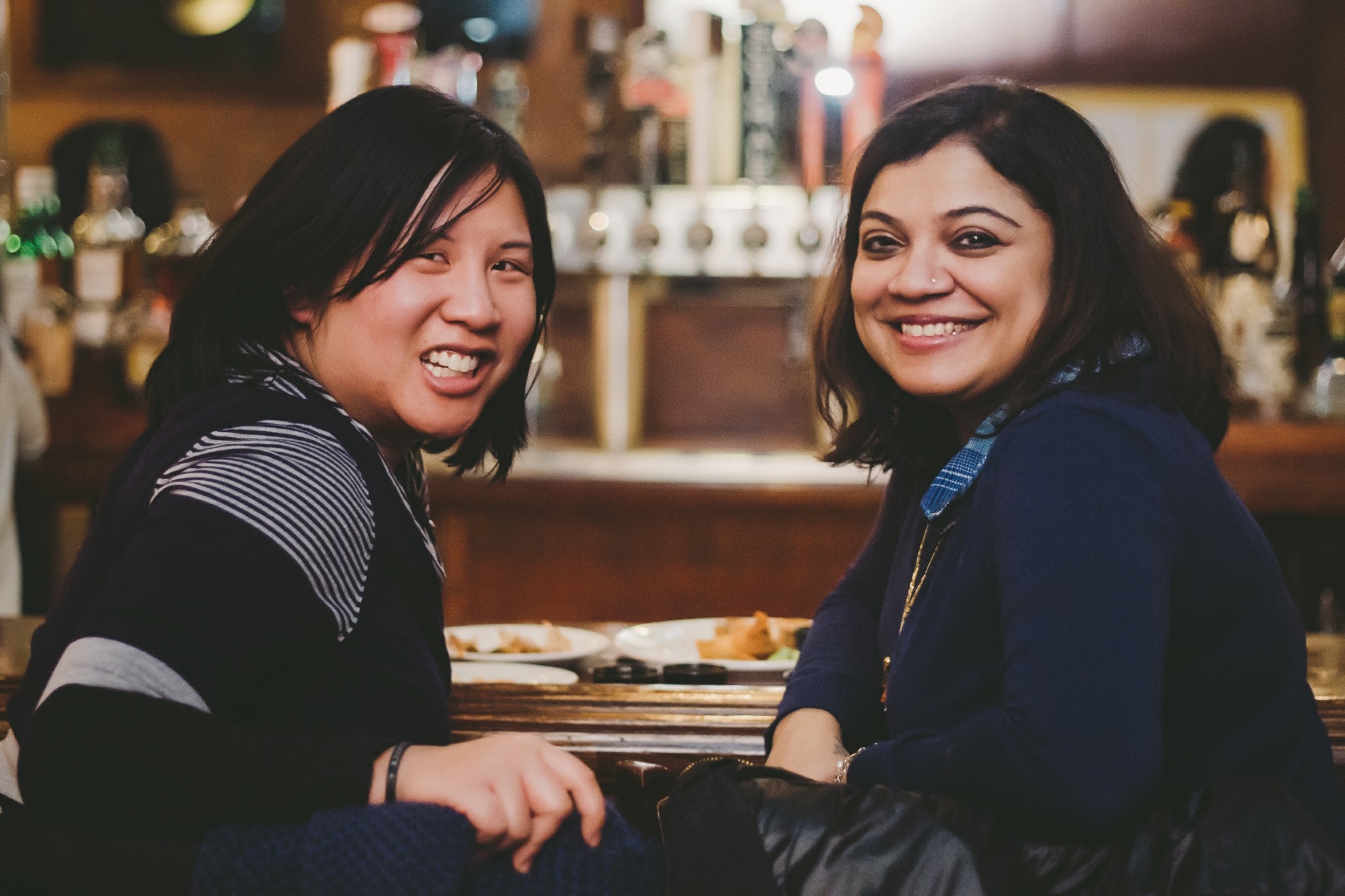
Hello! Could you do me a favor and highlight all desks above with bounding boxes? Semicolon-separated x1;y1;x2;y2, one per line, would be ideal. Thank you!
37;415;1345;627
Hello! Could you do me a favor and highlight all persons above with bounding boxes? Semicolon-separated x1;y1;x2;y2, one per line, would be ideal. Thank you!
1153;117;1291;405
0;318;52;619
769;82;1345;895
0;85;607;894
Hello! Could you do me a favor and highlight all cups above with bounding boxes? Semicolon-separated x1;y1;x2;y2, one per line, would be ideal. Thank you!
1303;630;1345;700
591;660;729;686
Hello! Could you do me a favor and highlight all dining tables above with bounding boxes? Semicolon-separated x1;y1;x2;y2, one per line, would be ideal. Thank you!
0;617;1345;801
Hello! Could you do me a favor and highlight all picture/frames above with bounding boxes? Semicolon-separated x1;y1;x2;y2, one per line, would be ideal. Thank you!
1031;84;1307;304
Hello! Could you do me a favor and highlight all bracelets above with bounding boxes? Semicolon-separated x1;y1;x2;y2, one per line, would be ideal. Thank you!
835;746;865;783
386;740;410;802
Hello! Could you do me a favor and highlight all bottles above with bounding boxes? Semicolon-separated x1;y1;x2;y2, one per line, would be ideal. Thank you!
586;217;648;455
0;160;215;400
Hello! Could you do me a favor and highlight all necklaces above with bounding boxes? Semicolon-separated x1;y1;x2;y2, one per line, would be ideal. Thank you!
882;524;942;706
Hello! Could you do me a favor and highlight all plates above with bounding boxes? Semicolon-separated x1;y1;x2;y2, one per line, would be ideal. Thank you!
442;622;610;667
450;660;580;686
617;618;824;669
